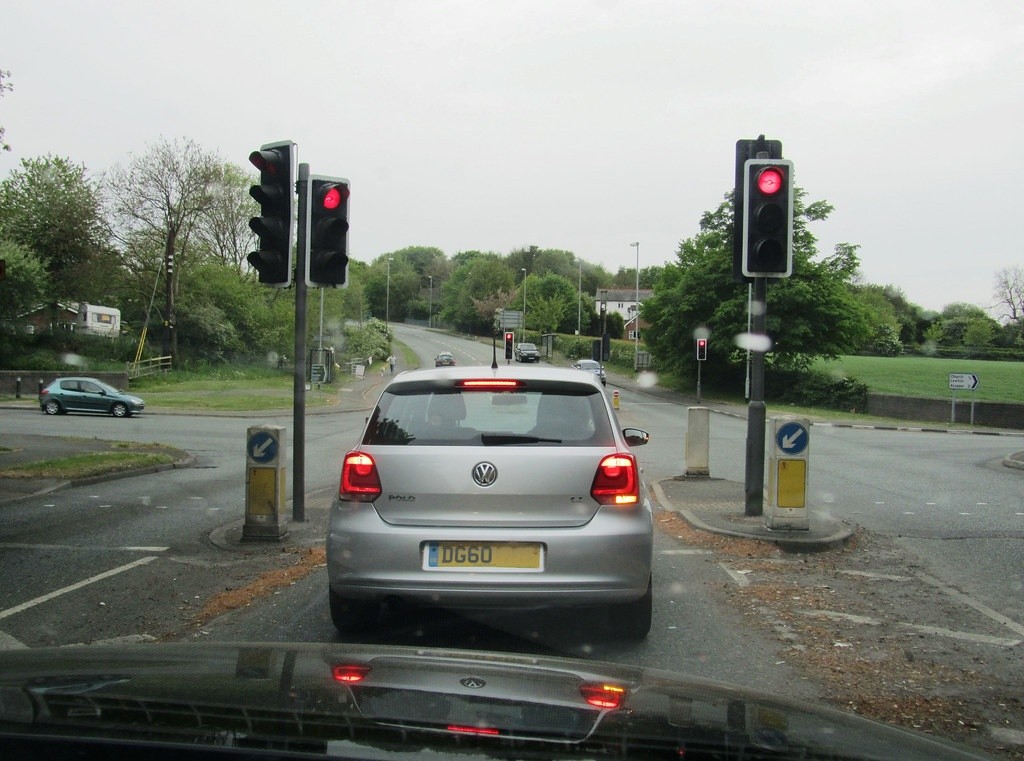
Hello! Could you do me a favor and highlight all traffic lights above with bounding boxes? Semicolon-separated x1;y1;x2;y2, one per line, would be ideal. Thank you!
305;172;352;290
742;159;793;279
696;339;707;361
247;139;293;288
504;331;514;361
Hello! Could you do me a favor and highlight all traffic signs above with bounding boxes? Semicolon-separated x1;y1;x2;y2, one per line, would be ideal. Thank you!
948;374;979;389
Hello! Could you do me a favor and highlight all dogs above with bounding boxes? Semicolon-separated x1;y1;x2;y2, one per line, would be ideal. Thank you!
380;367;385;377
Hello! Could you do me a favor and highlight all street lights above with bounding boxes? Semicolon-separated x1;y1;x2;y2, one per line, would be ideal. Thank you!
429;275;432;327
520;266;527;344
630;241;639;352
574;258;581;336
387;257;394;330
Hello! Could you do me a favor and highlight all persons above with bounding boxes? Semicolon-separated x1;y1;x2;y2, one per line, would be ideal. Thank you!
386;352;396;375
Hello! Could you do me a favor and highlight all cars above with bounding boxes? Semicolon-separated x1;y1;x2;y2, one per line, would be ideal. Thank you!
572;359;607;387
515;343;540;363
326;365;654;641
39;377;145;418
434;352;456;367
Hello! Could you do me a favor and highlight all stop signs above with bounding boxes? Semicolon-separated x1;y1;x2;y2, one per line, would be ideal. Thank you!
614;391;619;398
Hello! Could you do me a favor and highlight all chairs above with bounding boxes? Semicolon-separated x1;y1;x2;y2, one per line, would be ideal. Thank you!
528;391;593;442
409;392;477;444
